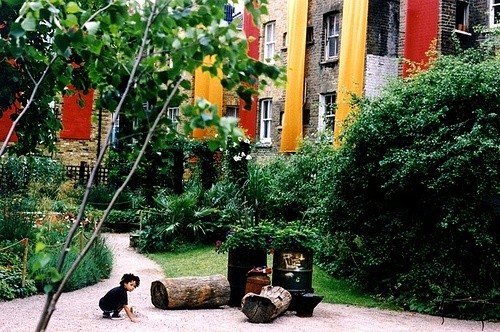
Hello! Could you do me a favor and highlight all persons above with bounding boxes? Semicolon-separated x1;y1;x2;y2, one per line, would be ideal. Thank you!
99;273;141;323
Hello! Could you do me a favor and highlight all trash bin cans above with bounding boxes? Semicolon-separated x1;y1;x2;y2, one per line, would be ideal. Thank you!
272;247;314;312
228;246;268;308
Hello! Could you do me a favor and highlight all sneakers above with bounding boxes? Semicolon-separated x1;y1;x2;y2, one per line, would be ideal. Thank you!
103;312;110;317
111;315;124;320
